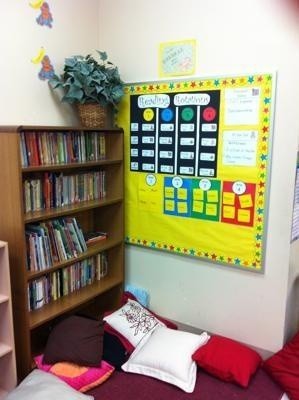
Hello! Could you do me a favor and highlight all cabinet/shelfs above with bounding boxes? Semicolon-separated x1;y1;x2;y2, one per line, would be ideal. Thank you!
0;240;18;400
0;125;125;387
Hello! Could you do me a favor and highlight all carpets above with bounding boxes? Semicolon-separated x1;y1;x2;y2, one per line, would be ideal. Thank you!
83;367;285;400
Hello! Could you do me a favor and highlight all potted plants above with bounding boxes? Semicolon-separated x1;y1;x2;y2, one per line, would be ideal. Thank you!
49;50;126;128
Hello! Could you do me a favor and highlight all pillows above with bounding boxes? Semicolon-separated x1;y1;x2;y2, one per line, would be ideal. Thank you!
4;290;299;400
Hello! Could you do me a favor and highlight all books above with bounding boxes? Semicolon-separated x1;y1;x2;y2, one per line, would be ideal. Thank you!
17;132;108;312
